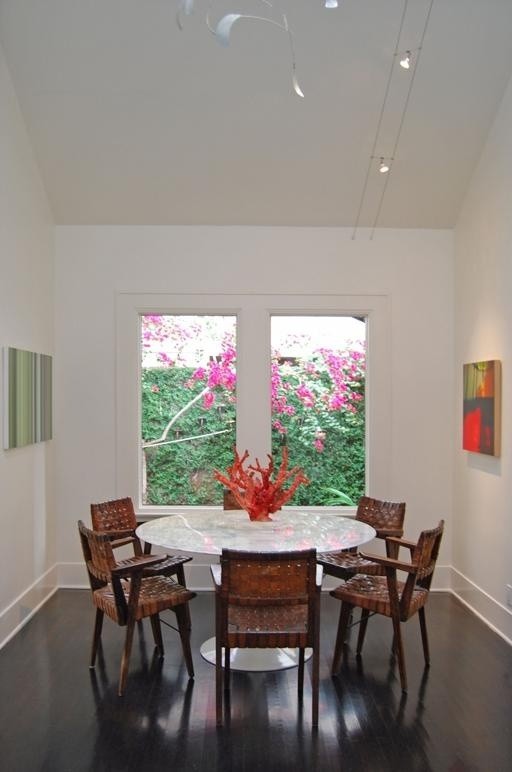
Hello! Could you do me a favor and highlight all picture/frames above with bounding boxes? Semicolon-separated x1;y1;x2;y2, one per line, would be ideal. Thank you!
460;360;504;458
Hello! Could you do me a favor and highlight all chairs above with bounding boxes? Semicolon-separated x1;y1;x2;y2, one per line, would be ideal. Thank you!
73;520;197;697
209;547;323;726
91;498;192;659
133;508;377;673
316;496;408;653
223;488;288;512
330;520;445;692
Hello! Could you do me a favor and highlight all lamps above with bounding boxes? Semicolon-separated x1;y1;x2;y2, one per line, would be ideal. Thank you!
400;50;414;69
375;156;391;174
